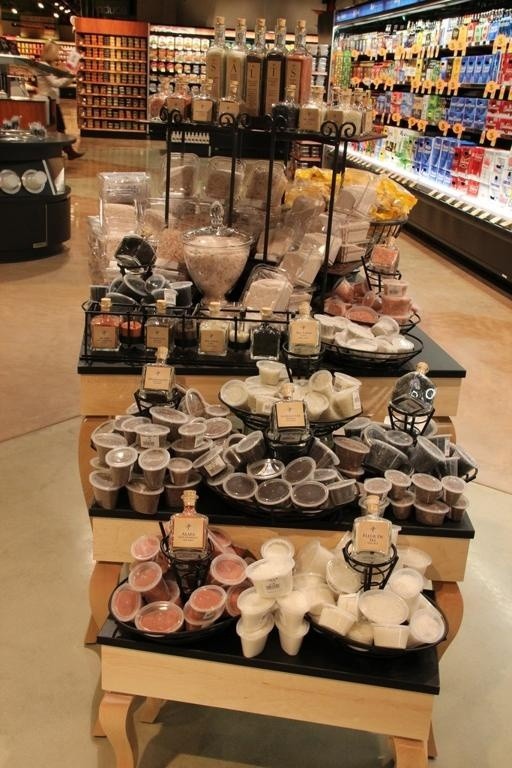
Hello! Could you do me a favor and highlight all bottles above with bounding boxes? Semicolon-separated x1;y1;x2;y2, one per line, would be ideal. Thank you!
84;226;399;360
147;14;375;135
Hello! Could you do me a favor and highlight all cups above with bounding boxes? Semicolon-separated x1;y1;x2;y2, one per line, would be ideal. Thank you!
89;358;479;658
378;279;414;358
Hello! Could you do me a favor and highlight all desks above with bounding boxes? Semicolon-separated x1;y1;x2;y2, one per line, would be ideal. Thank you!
86;486;474;760
96;569;441;767
0;128;76;261
76;318;469;647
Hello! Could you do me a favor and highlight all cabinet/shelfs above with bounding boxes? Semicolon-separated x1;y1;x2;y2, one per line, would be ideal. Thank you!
0;18;328;139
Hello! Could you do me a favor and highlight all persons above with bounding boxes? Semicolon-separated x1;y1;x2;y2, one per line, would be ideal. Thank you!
36;43;85;160
